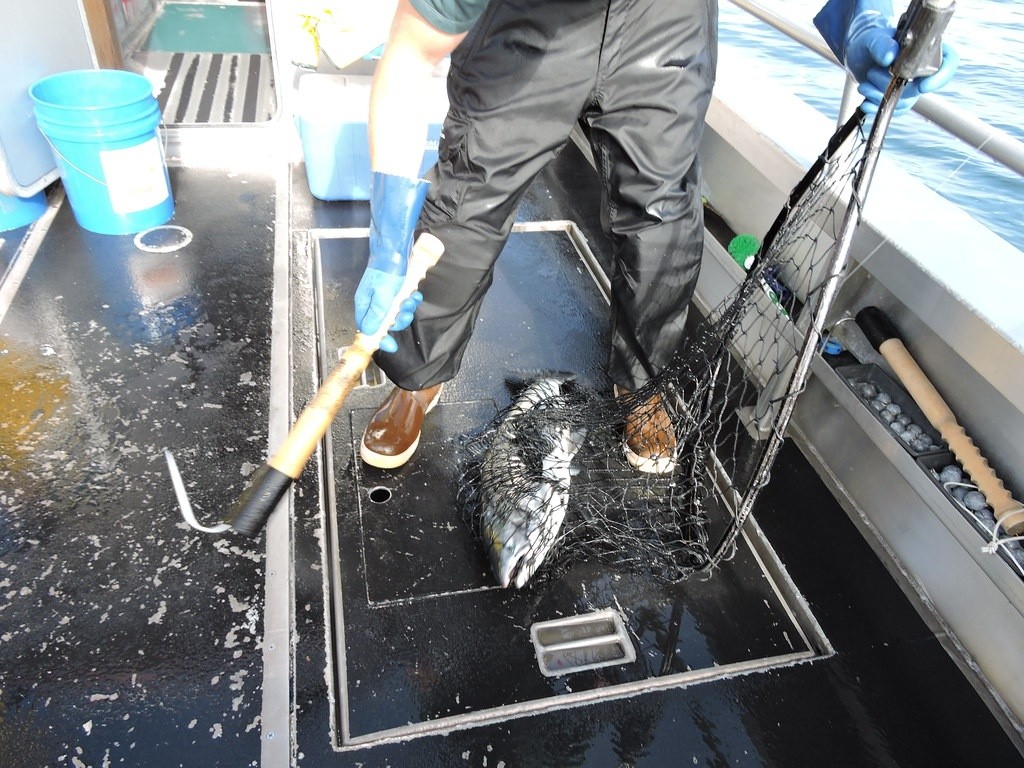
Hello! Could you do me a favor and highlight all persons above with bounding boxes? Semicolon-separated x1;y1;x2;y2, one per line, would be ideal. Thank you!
353;0;961;473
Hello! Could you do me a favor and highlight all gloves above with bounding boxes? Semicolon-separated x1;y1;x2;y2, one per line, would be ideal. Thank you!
812;0;958;114
350;169;430;353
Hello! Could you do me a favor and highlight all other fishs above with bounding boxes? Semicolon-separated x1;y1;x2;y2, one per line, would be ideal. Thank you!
479;366;588;588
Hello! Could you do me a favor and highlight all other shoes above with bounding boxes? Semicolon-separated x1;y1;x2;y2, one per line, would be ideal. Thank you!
612;381;677;474
360;382;444;467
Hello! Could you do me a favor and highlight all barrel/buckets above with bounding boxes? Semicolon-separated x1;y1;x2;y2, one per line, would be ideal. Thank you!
27;71;177;235
